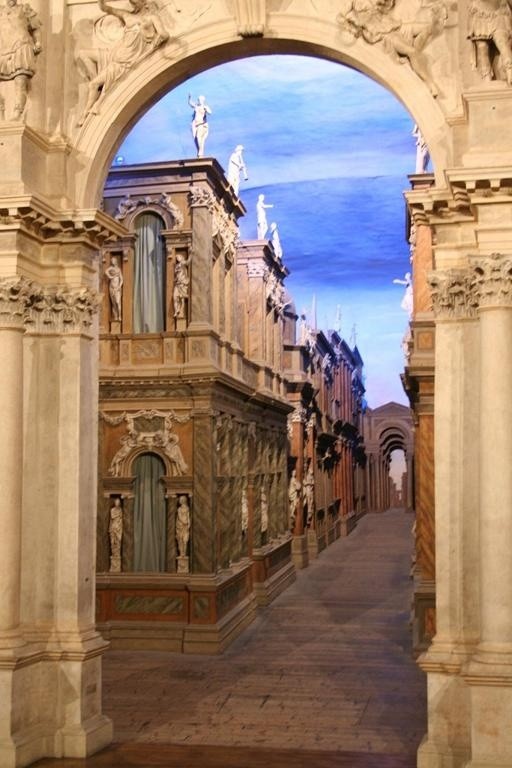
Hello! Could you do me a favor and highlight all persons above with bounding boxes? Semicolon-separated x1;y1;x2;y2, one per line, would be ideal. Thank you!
227;144;248;199
468;1;511;87
307;466;315;525
107;496;125;560
76;0;171;128
172;246;196;319
159;192;185;231
103;256;124;324
114;190;135;224
302;468;310;511
187;91;215;158
392;271;413;315
255;192;275;241
260;486;268;533
409;123;429;176
175;495;191;557
289;468;302;521
151;428;187;476
108;429;141;477
2;0;46;122
242;489;248;532
338;0;445;97
268;221;285;260
